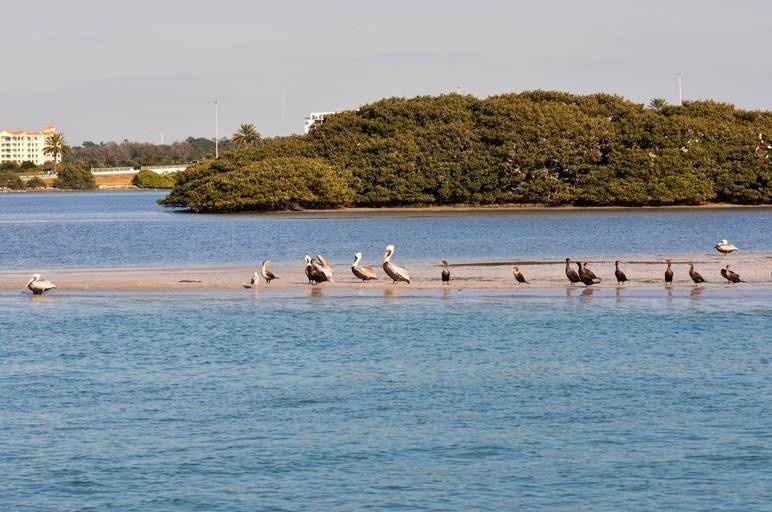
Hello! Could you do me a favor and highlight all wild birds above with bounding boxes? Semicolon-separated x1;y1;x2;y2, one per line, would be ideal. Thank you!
381;244;411;284
720;264;747;285
303;254;336;285
24;273;57;294
664;259;674;285
687;262;707;286
614;260;630;284
713;243;737;255
565;257;602;286
250;270;258;287
351;252;377;281
440;260;450;283
260;259;280;283
513;266;530;286
